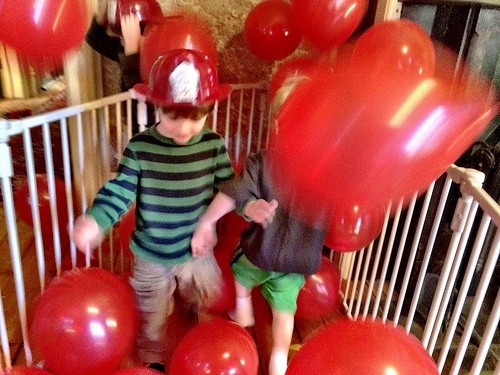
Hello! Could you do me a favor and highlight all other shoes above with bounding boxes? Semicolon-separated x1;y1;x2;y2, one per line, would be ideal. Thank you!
147;362;165;374
229;309;255;328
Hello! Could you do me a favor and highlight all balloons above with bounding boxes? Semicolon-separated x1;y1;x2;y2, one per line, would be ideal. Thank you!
0;0;499;375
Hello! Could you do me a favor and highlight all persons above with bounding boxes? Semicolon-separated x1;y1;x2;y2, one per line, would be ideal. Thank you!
191;75;330;375
74;48;278;372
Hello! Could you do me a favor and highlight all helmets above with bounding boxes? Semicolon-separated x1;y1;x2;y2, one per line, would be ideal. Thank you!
134;48;233;108
